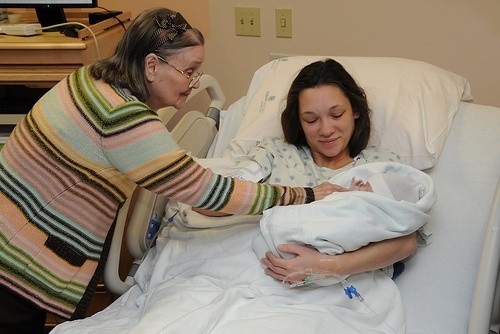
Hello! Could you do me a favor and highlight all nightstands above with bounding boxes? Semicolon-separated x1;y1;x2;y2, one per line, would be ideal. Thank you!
0;10;132;144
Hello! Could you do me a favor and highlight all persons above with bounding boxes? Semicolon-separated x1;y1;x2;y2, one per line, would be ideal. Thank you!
0;7;347;334
252;160;437;287
125;58;417;334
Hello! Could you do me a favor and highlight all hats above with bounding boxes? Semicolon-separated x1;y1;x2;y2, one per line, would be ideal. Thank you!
367;173;426;203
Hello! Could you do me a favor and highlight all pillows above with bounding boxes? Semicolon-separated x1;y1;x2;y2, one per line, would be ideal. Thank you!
233;56;473;170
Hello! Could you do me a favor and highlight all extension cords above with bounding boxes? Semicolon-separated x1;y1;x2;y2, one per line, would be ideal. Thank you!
0;23;42;36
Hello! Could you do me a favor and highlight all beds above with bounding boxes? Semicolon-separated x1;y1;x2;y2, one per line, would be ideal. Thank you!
49;53;500;334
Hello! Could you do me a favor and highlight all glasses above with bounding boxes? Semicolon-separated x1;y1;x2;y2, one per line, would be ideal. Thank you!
156;55;203;88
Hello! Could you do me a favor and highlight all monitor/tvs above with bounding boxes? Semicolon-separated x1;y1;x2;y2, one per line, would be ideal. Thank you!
0;0;98;32
